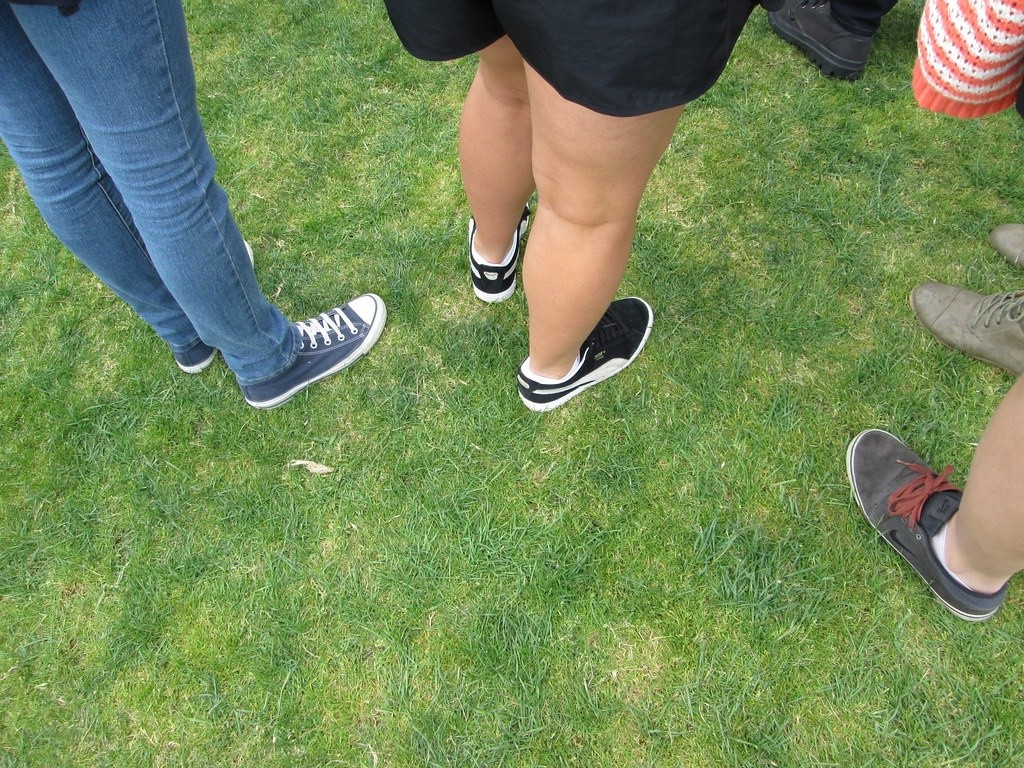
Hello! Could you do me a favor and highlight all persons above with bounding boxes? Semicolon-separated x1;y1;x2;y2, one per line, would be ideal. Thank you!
910;222;1024;376
0;0;389;408
768;0;894;79
383;0;782;414
911;0;1024;120
845;371;1024;621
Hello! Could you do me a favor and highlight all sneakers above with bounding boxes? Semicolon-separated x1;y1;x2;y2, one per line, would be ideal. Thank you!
466;198;528;304
171;240;256;374
768;0;872;80
846;429;1010;621
239;293;386;411
515;294;653;413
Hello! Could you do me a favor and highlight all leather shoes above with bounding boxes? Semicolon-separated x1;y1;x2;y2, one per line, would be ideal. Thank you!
990;220;1024;271
910;281;1024;372
